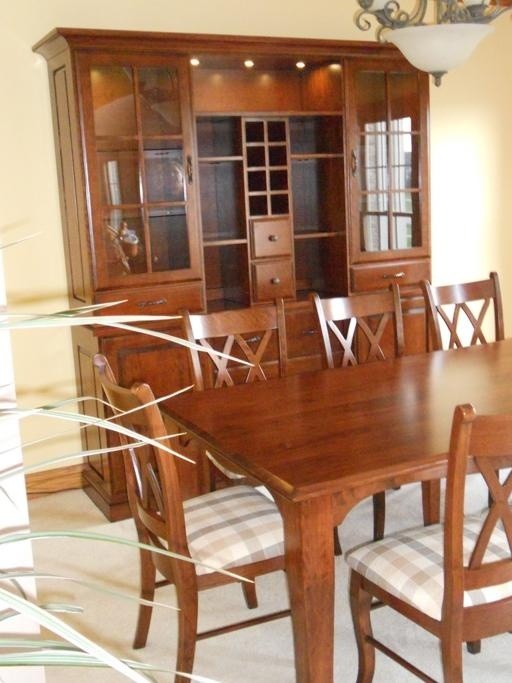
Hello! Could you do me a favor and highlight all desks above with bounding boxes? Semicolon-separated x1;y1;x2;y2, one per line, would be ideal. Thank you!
149;334;512;682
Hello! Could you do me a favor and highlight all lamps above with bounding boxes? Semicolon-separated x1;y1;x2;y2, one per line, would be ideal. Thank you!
356;0;512;87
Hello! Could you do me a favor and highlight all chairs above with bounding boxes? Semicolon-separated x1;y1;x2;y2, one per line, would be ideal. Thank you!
179;297;345;558
344;400;512;681
93;352;289;682
417;272;506;357
310;278;448;538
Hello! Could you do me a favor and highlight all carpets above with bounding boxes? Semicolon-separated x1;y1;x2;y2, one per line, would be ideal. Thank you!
34;466;512;682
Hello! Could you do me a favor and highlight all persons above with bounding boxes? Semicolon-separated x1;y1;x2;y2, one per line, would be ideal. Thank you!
159;160;190;269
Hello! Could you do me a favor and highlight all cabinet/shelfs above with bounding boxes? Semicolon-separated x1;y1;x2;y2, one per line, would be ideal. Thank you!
33;29;435;526
